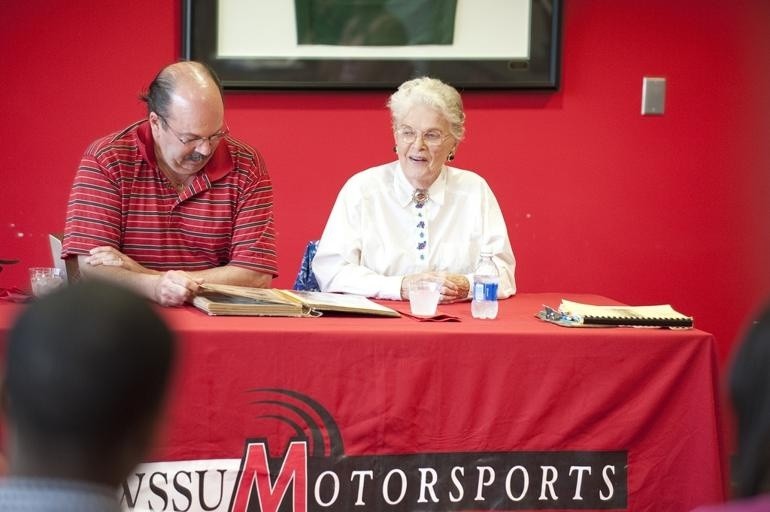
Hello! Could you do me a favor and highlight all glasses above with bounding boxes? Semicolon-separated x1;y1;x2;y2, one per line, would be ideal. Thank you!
155;110;229;148
397;127;453;146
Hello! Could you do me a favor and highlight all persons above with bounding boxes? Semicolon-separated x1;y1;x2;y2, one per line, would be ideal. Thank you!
685;301;770;512
0;271;180;512
312;81;516;305
59;60;277;307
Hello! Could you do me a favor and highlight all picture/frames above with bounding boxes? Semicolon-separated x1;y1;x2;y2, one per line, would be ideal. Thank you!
182;0;563;91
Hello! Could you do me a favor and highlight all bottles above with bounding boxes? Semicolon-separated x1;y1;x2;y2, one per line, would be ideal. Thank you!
471;246;500;319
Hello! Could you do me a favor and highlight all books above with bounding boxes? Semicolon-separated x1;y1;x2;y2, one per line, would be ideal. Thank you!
533;298;694;331
186;273;403;323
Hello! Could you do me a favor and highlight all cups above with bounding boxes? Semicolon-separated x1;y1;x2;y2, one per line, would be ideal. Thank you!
29;267;64;300
407;272;444;318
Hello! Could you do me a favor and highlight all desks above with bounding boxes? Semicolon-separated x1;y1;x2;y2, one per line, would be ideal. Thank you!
0;294;731;512
